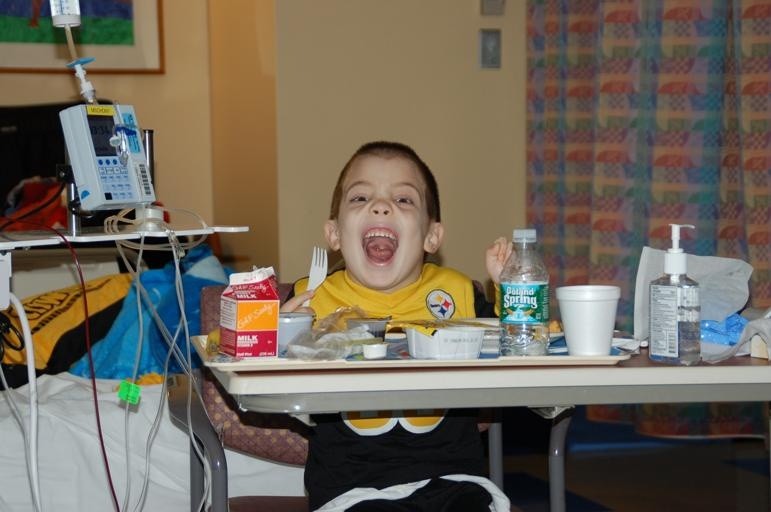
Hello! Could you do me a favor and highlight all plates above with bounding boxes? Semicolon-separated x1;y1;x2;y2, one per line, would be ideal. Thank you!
384;333;407;342
460;317;564;349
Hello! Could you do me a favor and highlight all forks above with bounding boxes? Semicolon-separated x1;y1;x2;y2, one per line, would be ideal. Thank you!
304;247;328;311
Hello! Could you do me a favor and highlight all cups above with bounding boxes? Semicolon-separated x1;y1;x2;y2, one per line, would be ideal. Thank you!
555;285;621;359
277;310;317;356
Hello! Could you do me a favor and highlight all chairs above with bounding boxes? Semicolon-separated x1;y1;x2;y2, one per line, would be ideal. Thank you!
168;283;505;512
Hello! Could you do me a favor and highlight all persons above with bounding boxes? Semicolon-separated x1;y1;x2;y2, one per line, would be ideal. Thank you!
274;139;565;512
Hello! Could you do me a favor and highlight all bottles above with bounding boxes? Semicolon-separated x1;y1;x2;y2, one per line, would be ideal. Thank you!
649;222;701;368
498;227;552;360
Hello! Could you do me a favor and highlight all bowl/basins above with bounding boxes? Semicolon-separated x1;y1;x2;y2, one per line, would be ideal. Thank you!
344;319;386;349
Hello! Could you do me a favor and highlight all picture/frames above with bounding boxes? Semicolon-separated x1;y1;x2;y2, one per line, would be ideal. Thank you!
0;0;166;74
